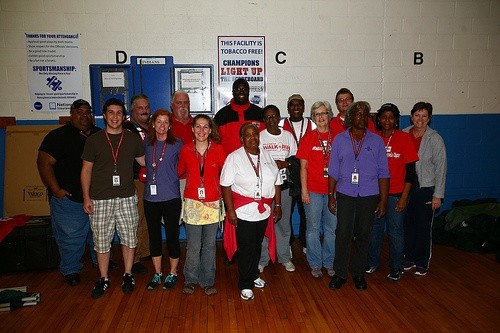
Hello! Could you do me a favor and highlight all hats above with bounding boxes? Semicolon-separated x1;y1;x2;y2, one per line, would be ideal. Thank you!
71;99;92;110
287;95;304;105
377;103;399;115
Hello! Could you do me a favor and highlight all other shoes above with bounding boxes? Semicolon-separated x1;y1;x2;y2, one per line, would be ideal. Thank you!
254;277;267;288
311;266;323;278
204;286;218;296
132;263;148;274
240;288;254;300
224;256;236;265
282;261;296;271
258;264;268;273
181;283;196;295
94;261;119;271
65;273;80;285
161;272;178;290
326;266;336;276
146;271;163;290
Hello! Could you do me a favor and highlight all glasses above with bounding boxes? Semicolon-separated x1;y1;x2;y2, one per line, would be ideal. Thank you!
314;110;330;116
336;97;353;103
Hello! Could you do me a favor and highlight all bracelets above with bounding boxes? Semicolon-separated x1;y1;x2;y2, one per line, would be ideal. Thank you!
275;204;280;207
329;192;333;194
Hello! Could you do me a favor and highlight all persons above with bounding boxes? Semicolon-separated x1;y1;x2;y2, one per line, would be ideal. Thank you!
200;190;203;194
114;178;118;182
214;79;264;266
353;176;357;180
219;123;283;301
255;88;448;291
122;94;152;276
176;114;225;296
37;100;117;285
160;91;194;226
256;192;260;196
81;98;144;298
139;110;185;290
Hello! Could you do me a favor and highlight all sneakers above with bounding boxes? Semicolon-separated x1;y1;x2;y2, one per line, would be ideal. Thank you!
402;258;416;271
386;268;405;281
412;261;430;276
91;277;112;298
365;262;380;273
122;272;137;294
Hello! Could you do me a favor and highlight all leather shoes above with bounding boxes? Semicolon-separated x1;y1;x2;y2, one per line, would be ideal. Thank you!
329;273;347;289
352;273;368;289
